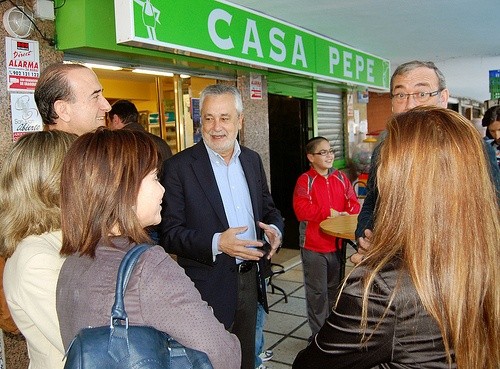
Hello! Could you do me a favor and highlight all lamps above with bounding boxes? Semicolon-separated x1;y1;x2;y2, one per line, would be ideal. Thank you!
3;6;36;39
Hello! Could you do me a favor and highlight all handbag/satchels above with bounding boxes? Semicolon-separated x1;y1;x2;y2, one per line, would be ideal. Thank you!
61;242;214;369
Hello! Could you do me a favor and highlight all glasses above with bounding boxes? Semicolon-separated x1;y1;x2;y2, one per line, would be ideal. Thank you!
390;88;446;103
306;148;335;156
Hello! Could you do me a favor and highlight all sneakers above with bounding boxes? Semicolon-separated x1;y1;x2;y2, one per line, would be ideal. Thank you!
255;363;270;369
259;348;273;362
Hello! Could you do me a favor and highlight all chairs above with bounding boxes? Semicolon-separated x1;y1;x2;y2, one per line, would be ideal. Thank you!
267;263;287;304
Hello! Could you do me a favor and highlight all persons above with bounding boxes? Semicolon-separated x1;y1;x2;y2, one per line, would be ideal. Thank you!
248;264;275;369
107;98;173;244
0;131;85;369
34;64;112;136
296;105;500;369
351;60;500;246
481;107;500;197
161;83;285;369
293;136;360;341
55;125;242;368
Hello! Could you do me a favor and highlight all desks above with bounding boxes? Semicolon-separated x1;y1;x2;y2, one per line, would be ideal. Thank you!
320;214;358;282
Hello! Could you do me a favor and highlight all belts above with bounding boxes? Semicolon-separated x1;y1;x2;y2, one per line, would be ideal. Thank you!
237;260;257;274
